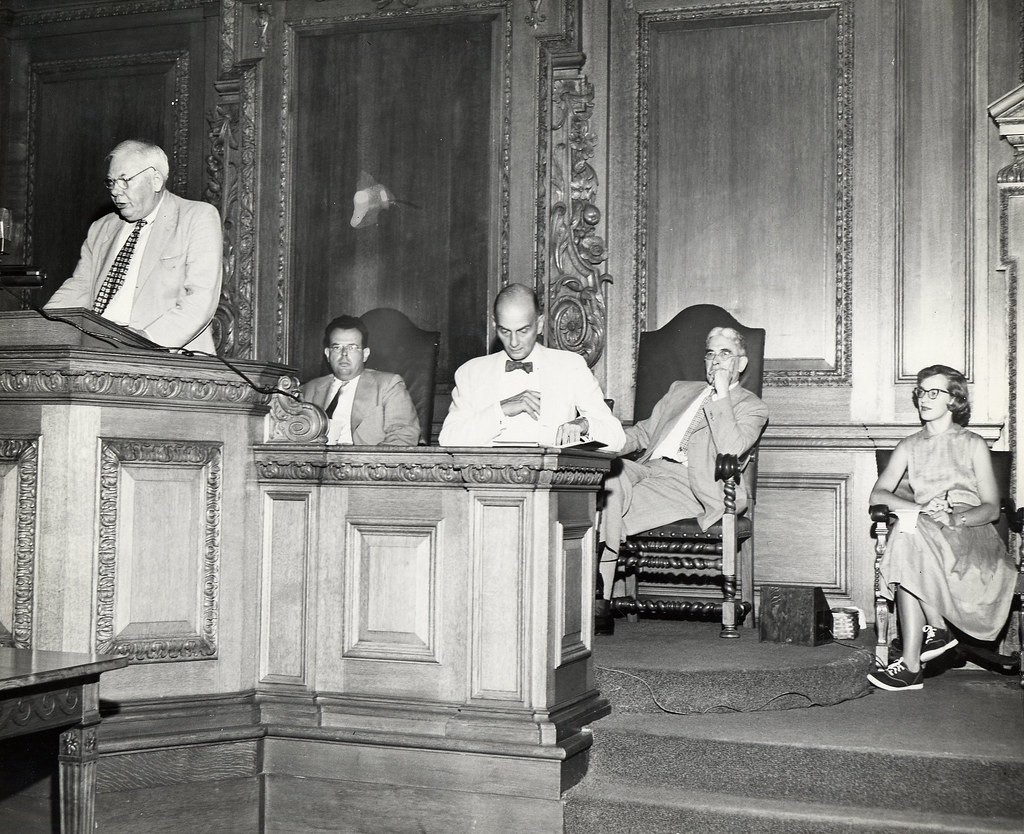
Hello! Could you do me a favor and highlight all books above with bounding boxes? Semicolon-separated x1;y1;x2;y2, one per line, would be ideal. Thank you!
493;440;608;448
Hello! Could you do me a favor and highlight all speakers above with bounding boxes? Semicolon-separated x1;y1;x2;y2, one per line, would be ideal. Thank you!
757;584;834;646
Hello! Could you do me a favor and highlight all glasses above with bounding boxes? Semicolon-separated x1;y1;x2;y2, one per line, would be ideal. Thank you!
103;166;156;190
705;351;741;361
915;387;950;399
330;345;362;353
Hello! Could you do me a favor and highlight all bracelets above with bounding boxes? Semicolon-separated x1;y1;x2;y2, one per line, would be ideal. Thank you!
959;513;966;525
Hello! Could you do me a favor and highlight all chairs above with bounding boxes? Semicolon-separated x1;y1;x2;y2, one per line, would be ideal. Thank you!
871;449;1024;689
358;306;442;446
608;304;766;639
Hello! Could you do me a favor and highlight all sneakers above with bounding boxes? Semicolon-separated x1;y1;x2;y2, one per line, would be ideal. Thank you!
921;625;958;662
867;657;924;691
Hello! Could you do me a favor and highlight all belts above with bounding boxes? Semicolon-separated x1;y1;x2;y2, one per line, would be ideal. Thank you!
661;456;682;465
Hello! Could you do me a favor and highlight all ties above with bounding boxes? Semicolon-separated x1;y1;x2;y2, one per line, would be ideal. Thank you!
91;219;147;316
324;383;347;420
677;389;717;456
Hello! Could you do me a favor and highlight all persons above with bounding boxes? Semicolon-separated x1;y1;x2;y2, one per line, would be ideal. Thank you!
595;326;769;635
41;140;223;357
866;365;1016;692
302;315;420;447
439;283;627;452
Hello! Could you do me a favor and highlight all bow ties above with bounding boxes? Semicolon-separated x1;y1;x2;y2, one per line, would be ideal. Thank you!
505;359;533;374
